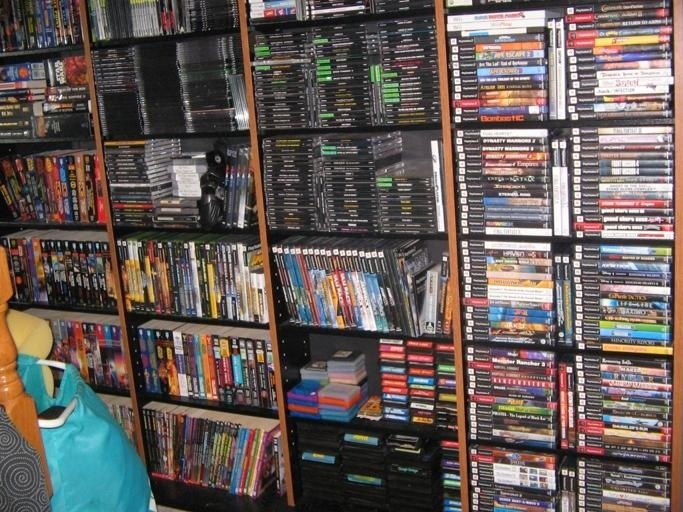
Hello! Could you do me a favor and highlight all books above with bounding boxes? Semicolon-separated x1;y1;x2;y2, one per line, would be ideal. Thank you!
249;1;463;511
1;1;138;456
446;1;674;511
130;1;285;499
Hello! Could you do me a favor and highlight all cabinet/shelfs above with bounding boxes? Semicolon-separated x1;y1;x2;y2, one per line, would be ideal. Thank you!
241;0;467;508
81;0;290;502
1;1;144;478
440;1;678;507
